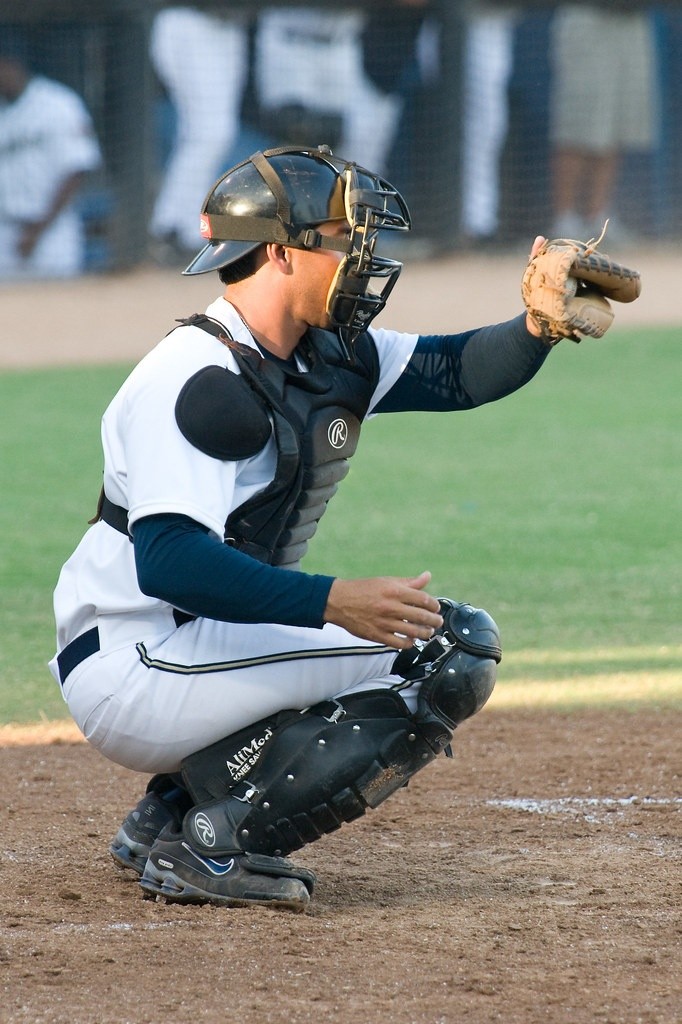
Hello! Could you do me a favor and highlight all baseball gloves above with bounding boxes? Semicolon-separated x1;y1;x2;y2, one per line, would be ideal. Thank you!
521;238;641;346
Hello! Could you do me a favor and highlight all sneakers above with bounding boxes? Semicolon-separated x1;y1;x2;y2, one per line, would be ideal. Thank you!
110;788;177;875
142;829;318;910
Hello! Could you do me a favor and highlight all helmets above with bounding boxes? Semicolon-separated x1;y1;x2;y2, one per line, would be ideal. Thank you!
181;146;387;279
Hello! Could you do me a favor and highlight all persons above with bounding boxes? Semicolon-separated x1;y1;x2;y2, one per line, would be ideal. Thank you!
47;146;641;913
0;0;662;280
